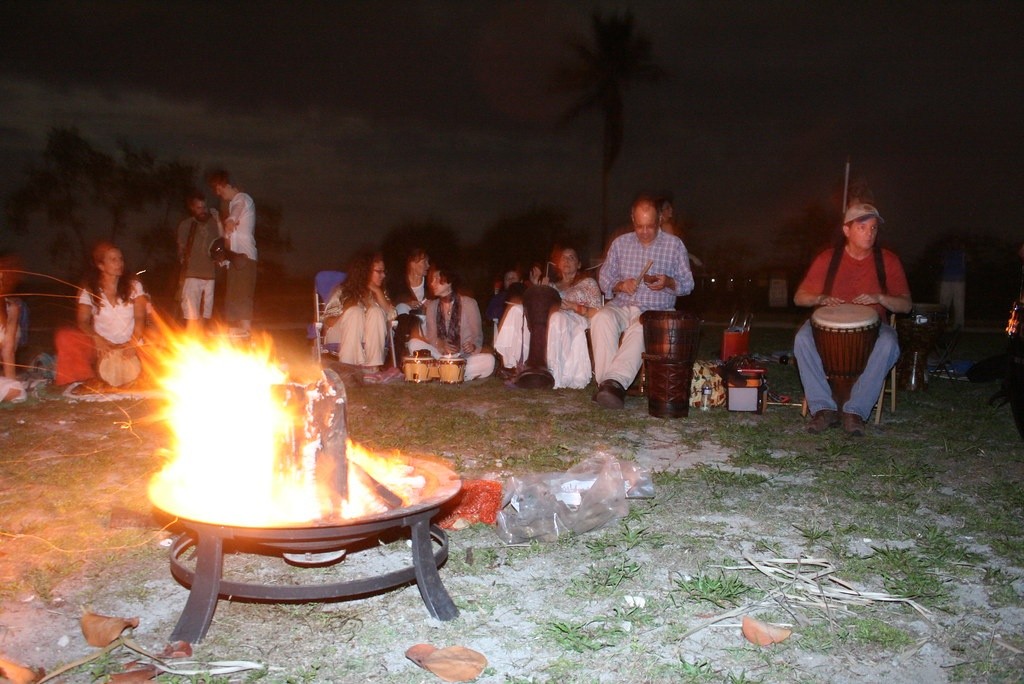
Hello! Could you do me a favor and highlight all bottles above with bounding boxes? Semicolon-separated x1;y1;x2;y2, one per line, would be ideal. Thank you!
700;376;712;411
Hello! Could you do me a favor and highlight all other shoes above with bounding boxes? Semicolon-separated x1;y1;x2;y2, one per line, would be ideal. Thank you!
362;363;380;385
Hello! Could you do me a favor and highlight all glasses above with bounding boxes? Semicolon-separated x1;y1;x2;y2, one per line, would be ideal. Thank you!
371;268;388;276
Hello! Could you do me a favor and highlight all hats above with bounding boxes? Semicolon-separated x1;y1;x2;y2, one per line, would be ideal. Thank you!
843;204;884;225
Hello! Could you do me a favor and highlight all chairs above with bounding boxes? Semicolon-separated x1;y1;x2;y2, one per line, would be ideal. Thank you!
800;310;899;426
306;270;398;369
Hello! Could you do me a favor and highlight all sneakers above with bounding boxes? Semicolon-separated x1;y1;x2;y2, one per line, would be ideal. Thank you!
590;378;625;409
841;411;865;435
807;409;841;435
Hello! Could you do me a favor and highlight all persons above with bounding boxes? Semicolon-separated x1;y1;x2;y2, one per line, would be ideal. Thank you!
793;204;913;435
175;169;258;326
487;243;602;389
0;257;29;404
939;239;967;331
55;240;151;395
588;193;694;408
323;242;495;385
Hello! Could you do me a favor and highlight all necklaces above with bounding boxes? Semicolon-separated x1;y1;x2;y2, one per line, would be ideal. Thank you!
444;303;451;315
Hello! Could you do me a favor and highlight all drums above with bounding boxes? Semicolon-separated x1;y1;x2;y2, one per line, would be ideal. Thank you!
435;357;469;386
516;284;562;391
894;302;949;392
810;303;882;412
403;356;435;383
639;310;704;418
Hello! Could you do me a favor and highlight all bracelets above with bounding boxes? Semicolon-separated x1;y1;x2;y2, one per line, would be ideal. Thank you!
436;338;439;344
577;304;584;315
